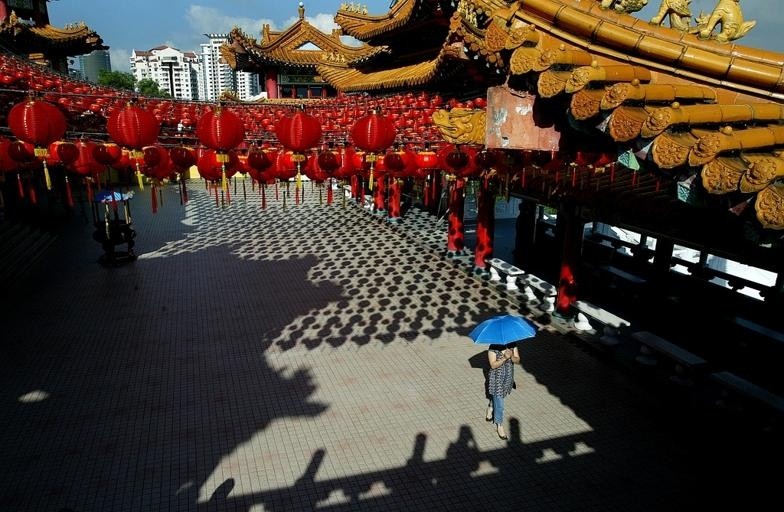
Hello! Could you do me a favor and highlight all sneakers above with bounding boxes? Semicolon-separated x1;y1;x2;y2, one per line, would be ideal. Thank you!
486;413;493;421
497;428;507;440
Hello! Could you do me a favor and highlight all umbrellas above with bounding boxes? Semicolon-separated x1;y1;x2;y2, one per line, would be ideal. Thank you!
467;311;536;346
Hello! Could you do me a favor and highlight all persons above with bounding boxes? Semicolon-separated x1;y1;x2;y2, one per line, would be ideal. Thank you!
484;343;522;440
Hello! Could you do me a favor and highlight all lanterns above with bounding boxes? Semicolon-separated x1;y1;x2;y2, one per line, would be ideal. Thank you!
1;43;481;218
